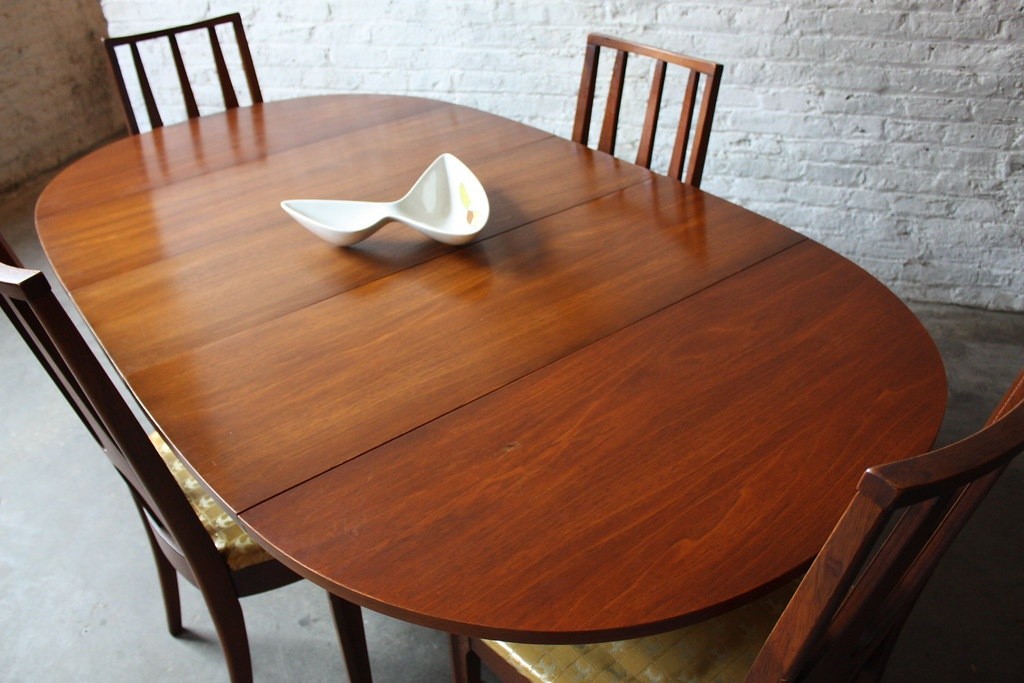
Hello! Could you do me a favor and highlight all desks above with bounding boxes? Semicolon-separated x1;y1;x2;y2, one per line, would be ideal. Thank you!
36;91;948;683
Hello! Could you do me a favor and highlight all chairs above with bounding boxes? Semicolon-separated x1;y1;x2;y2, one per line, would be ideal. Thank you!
570;28;725;192
0;233;375;683
447;361;1024;683
98;10;264;134
